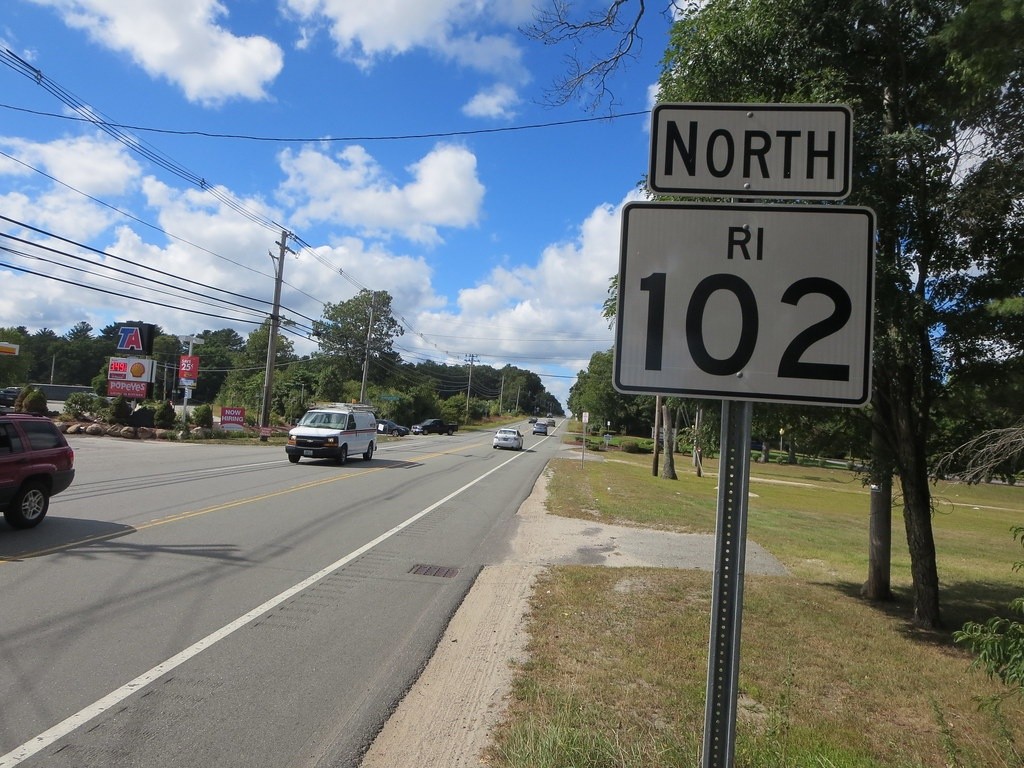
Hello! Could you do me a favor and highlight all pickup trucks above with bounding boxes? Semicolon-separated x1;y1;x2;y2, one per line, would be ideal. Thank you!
411;419;458;435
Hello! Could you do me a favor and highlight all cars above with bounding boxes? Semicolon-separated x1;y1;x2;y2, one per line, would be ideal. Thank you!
492;429;523;451
547;413;553;418
0;387;23;407
529;417;538;423
375;419;410;438
546;419;556;427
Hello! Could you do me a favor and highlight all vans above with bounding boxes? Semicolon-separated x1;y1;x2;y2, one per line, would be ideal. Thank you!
285;408;377;465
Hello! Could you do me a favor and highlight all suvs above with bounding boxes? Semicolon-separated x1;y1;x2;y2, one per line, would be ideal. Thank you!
0;411;74;529
532;422;547;436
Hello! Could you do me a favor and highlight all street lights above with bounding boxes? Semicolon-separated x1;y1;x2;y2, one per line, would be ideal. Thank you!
179;334;205;422
